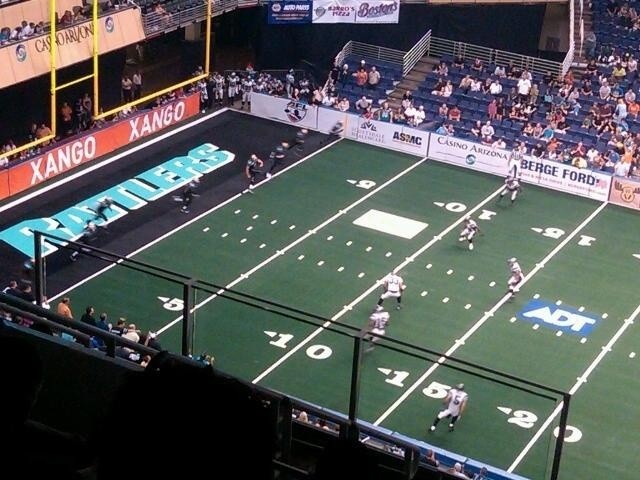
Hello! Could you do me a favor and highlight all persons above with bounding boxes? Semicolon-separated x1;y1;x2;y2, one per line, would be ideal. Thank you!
245;154;264;194
1;1;129;42
19;257;37;285
366;305;390;352
605;0;640;31
1;281;216;371
583;30;597;61
68;219;110;261
93;196;114;230
497;177;522;206
180;181;196;214
458;216;485;250
188;62;311;112
290;411;487;480
428;383;469;433
505;257;524;298
143;1;173;24
376;269;408;312
312;61;393;124
1;71;186;168
393;47;639;179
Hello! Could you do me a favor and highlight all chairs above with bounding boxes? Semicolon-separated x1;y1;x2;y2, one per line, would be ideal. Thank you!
402;2;640;182
331;53;401;117
